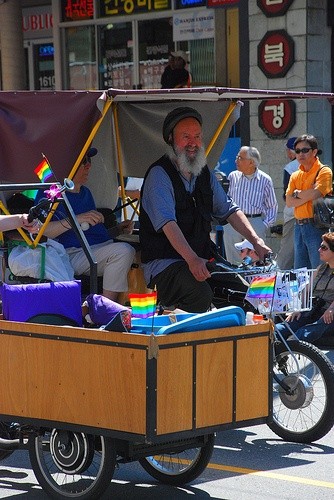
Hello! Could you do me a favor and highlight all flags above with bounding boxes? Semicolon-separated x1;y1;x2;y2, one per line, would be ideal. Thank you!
129;290;158;315
34;157;53;183
246;275;276;299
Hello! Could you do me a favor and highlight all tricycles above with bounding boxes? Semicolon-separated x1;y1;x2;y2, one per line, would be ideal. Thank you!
0;280;274;500
0;86;334;443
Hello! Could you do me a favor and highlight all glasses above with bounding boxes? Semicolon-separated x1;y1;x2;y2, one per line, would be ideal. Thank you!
320;243;328;251
78;156;91;167
236;155;252;161
294;148;313;154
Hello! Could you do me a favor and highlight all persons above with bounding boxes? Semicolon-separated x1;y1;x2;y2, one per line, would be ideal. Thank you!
34;146;135;303
0;189;48;266
161;50;189;89
285;135;332;272
272;232;334;352
234;237;291;322
275;136;302;270
138;107;273;313
219;145;277;266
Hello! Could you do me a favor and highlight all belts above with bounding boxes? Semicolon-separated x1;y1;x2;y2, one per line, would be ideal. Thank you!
245;214;262;218
295;218;313;225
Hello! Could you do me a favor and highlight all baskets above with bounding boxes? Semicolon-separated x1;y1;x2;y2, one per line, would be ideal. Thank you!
246;269;317;314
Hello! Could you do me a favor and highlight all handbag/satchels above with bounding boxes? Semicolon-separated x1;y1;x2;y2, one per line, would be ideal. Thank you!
8;237;75;283
313;165;334;228
302;295;326;323
118;261;148;306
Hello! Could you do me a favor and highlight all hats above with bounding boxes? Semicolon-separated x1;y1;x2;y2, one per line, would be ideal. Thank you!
234;237;265;252
286;137;296;150
163;106;203;145
86;148;98;157
